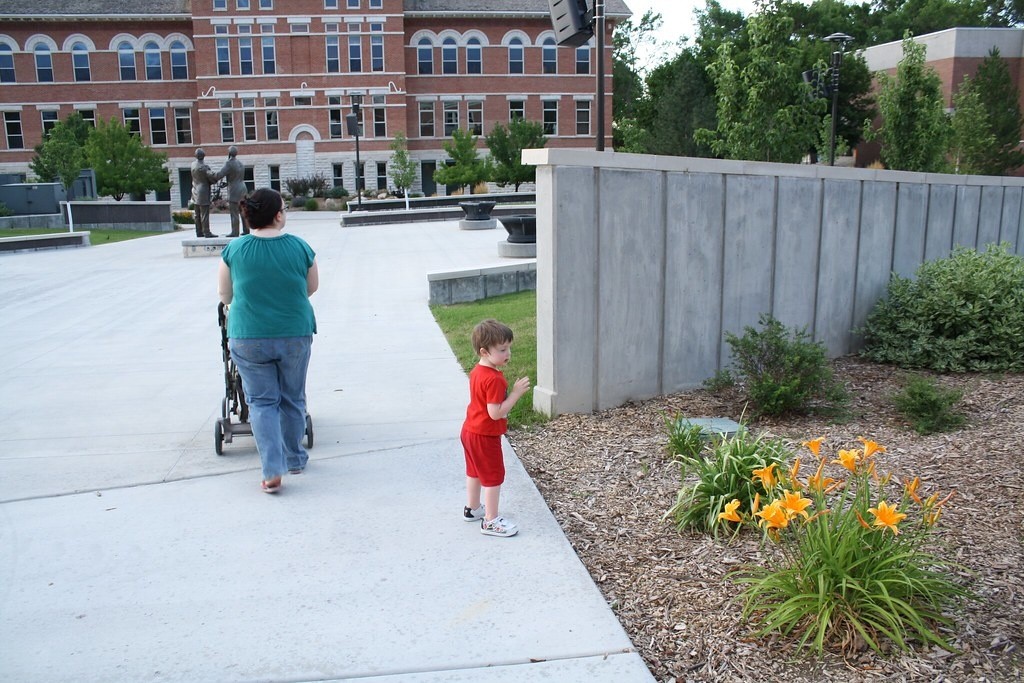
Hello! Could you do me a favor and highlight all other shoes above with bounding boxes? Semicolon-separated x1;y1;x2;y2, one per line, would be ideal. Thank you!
260;478;281;493
288;469;302;474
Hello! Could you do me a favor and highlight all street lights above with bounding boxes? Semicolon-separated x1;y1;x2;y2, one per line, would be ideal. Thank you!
822;32;856;166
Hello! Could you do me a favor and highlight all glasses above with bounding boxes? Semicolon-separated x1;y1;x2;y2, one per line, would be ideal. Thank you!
281;205;289;211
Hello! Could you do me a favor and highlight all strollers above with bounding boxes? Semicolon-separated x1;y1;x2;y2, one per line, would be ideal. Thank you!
214;303;314;456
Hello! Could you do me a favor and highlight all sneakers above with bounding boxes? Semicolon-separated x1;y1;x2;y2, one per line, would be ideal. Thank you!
480;516;518;537
464;504;485;521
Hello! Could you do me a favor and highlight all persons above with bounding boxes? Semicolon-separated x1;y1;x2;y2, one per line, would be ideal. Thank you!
192;146;251;238
218;188;318;492
460;319;530;536
